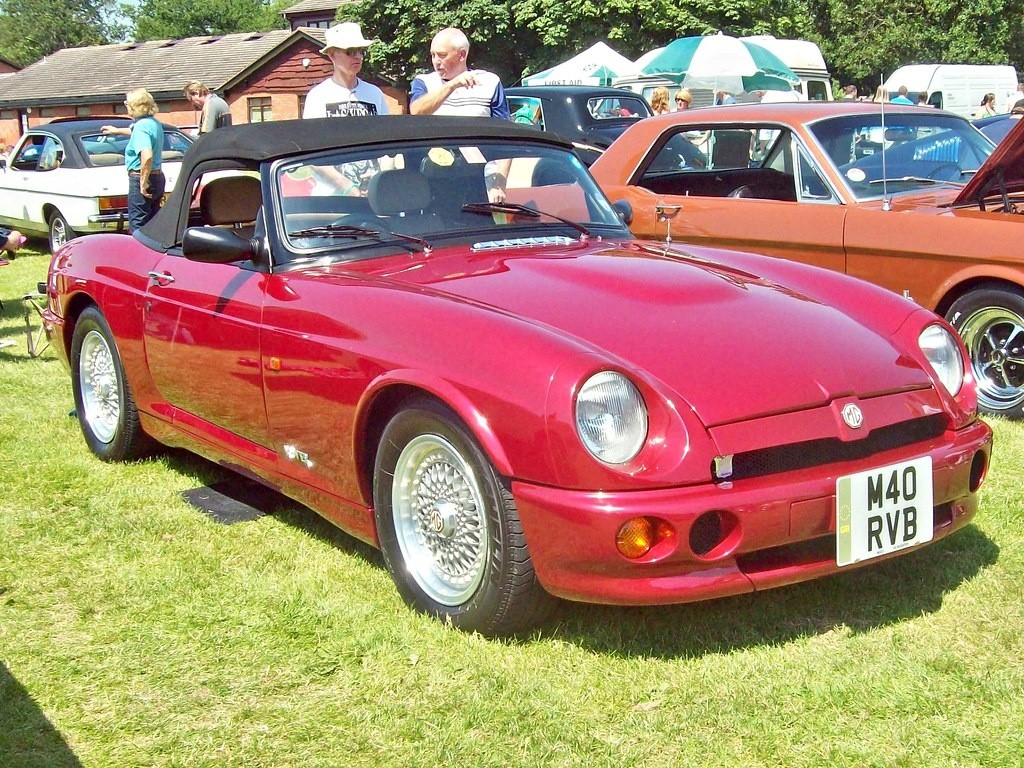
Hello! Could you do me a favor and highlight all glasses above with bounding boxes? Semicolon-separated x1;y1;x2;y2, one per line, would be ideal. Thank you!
677;98;686;102
336;48;367;57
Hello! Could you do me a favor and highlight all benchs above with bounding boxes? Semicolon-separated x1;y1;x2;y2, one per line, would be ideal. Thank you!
639;167;792;200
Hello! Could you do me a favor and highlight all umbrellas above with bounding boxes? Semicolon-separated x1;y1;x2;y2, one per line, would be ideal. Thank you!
640;30;803;170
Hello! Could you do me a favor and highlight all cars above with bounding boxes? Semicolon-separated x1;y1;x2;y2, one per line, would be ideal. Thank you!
0;115;201;256
501;86;1024;422
43;115;993;640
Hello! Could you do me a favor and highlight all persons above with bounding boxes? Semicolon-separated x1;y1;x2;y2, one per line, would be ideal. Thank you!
0;228;21;250
711;89;736;106
1006;82;1024;112
650;86;670;117
875;85;889;103
918;92;927;106
844;85;857;98
976;93;997;119
674;89;711;146
183;80;233;136
410;28;512;203
755;90;803;160
100;88;166;232
891;86;913;103
302;23;395;197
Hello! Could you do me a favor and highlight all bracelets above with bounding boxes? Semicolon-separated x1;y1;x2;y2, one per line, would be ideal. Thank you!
343;183;354;195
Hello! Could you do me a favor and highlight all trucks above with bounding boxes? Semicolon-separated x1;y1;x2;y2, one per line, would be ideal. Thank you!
604;36;834;118
885;65;1018;124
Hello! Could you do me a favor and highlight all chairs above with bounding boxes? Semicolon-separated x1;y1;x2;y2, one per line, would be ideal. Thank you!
200;174;263;237
162;151;184;159
89;153;124;165
370;169;445;234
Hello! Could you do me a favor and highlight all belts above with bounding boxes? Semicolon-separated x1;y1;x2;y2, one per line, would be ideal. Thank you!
131;170;162;175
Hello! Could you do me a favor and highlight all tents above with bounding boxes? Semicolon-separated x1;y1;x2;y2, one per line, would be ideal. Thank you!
522;41;633;87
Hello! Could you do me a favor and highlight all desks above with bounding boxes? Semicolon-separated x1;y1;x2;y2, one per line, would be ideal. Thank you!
21;290;51;358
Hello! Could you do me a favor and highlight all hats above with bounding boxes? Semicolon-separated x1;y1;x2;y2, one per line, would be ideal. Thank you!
319;23;379;55
